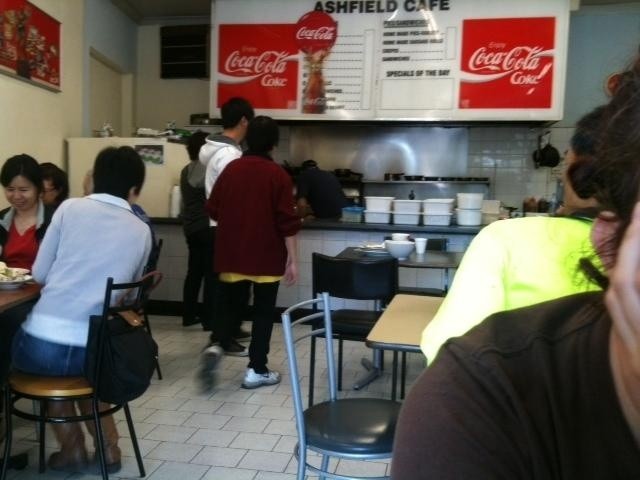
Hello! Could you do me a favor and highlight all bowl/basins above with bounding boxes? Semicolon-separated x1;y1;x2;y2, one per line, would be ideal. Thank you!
385;242;414;260
455;192;500;226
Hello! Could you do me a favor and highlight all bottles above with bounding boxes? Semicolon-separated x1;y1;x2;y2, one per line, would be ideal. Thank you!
524;193;558;213
166;120;176;134
302;64;326;115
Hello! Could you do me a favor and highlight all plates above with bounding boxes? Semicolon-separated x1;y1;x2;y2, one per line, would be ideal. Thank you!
1;266;31;288
406;175;488;182
335;168;351;173
353;242;389;257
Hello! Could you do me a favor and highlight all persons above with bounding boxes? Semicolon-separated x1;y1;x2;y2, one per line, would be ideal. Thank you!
1;151;55;273
179;131;216;331
79;166;150;225
197;115;314;389
417;102;616;367
37;160;66;205
7;141;159;474
197;95;252;360
388;60;640;478
297;160;351;218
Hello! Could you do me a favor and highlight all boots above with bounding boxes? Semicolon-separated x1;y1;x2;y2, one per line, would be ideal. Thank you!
48;397;121;474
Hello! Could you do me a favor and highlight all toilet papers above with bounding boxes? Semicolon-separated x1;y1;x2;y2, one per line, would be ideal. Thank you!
169;186;182;219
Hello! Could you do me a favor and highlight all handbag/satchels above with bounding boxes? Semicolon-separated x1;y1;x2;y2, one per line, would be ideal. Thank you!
82;303;159;404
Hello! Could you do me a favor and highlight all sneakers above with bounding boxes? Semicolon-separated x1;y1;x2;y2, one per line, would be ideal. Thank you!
242;367;281;389
193;344;224;394
182;318;211;332
223;329;251;356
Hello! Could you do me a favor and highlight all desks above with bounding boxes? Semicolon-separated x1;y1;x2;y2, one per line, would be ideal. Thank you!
329;244;468;391
1;280;48;473
361;291;447;403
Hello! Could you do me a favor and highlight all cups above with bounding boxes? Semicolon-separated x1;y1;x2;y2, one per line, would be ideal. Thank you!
384;173;390;181
414;238;427;256
393;234;410;241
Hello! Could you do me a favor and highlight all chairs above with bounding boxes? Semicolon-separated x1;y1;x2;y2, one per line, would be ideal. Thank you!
1;268;163;479
302;251;409;411
278;289;407;480
120;236;165;384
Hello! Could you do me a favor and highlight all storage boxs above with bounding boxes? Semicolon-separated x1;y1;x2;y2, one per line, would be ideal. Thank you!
339;194;456;229
